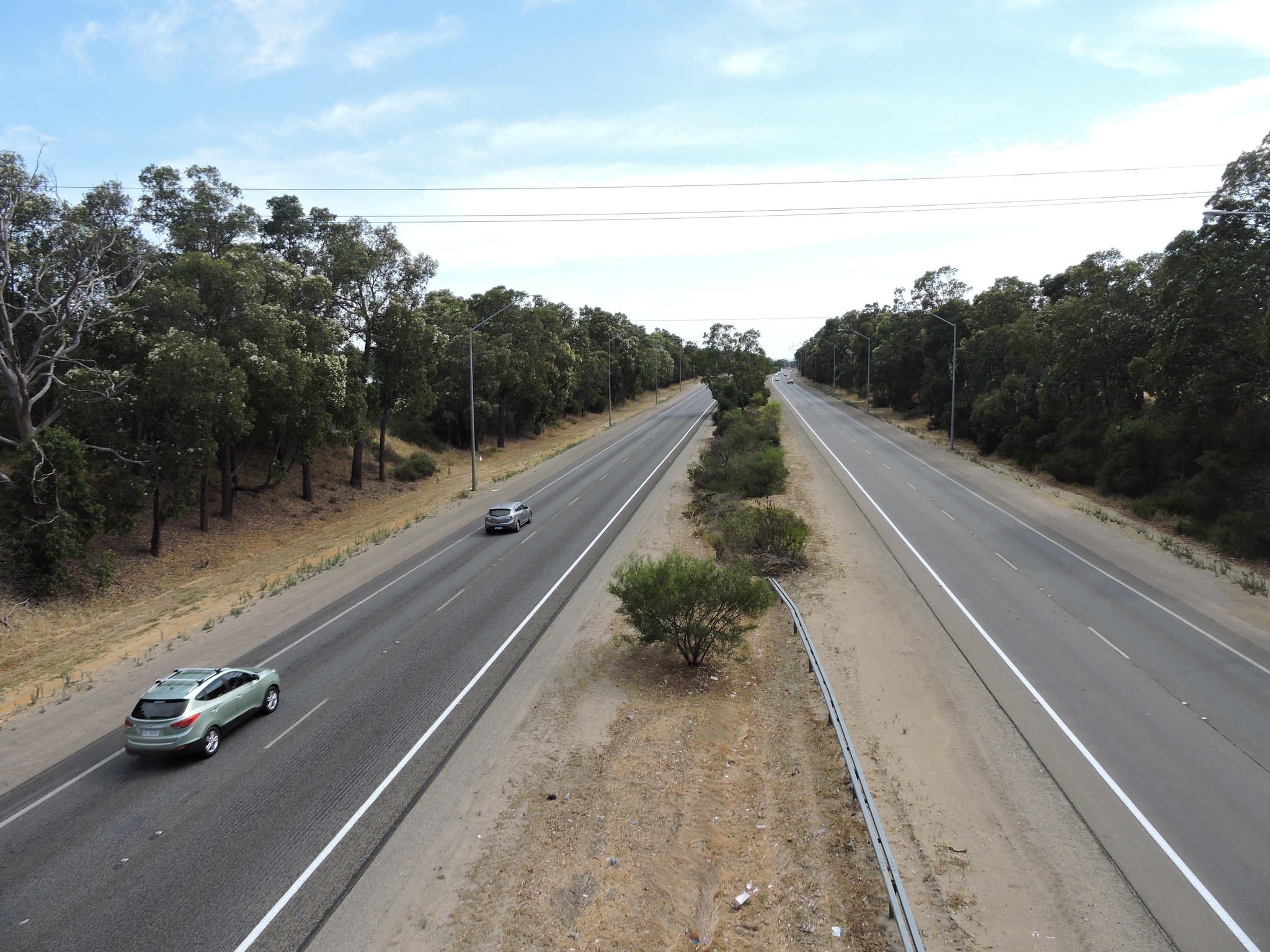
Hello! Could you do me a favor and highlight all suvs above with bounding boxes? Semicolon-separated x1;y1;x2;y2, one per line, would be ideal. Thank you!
124;668;281;759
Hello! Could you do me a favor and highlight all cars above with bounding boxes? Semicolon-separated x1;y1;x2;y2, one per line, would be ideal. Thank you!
781;371;784;373
775;373;778;376
777;372;781;375
787;378;794;384
775;377;779;381
789;370;793;373
485;501;532;535
784;374;787;376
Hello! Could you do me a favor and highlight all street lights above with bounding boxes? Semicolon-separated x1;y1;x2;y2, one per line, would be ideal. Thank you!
900;308;956;450
789;345;806;382
799;342;816;384
813;338;835;396
469;294;543;489
656;338;684;403
680;341;698;392
609;327;646;425
838;329;870;412
